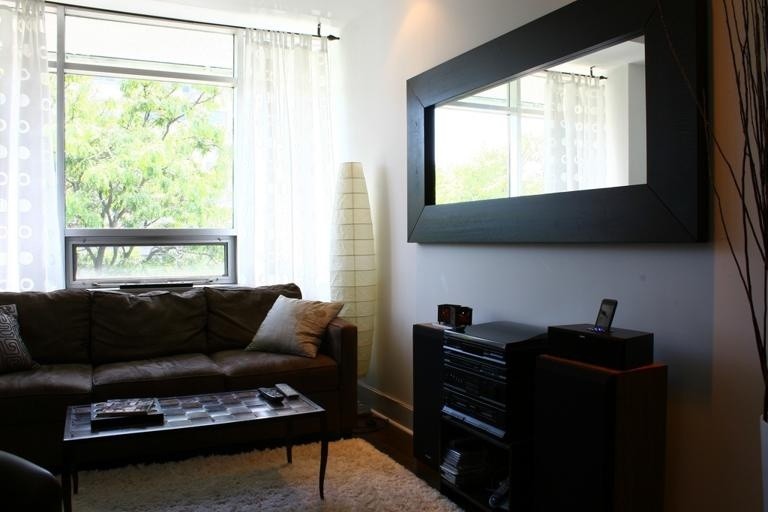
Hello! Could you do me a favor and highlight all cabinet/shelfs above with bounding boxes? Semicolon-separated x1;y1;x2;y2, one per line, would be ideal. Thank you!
435;320;548;512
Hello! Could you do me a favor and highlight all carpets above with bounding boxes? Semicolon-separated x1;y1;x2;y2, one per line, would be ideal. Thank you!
49;436;466;512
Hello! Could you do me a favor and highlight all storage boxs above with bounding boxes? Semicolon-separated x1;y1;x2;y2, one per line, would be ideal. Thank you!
540;320;655;371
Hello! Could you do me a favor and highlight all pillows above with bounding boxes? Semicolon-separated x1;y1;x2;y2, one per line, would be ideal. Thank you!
246;289;345;361
0;306;42;376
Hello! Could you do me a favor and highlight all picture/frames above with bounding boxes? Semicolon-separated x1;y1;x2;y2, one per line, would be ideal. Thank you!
592;294;618;331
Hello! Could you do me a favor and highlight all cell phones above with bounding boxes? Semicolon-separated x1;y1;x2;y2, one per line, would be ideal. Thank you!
593;298;620;333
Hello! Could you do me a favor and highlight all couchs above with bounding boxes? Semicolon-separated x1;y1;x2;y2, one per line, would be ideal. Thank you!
0;449;72;510
0;285;360;463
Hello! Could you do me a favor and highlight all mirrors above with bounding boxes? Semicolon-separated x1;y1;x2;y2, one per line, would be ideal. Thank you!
404;2;714;247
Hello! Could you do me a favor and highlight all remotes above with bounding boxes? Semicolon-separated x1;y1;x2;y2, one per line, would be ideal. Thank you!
275;383;299;400
257;387;284;402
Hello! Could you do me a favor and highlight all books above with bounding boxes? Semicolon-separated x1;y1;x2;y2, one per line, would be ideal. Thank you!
97;397;153;417
